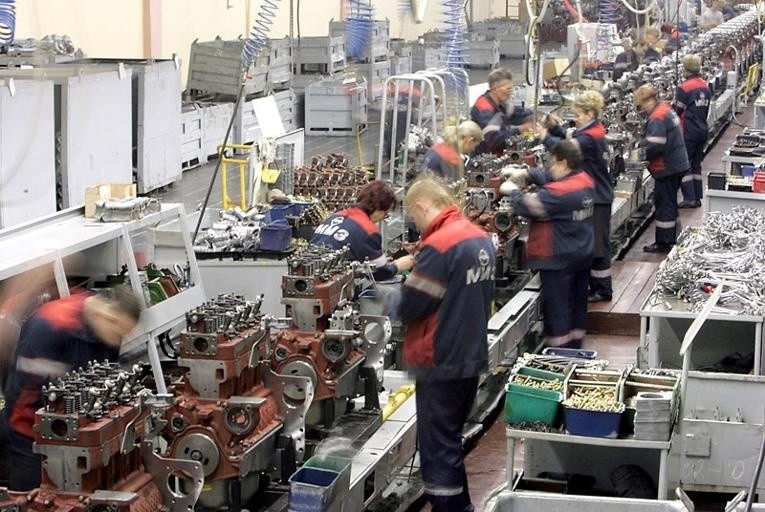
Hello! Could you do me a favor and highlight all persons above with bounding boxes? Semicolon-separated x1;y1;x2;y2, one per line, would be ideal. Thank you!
369;179;497;511
470;67;537;158
674;54;712;208
3;283;142;492
612;22;673;81
308;180;415;281
499;139;595;348
536;89;619;303
634;84;691;252
409;120;485;243
701;0;724;33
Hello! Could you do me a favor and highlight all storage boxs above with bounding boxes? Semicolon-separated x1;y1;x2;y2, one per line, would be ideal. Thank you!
358;289;393;316
303;454;352;494
708;162;765;200
287;467;340;512
543;58;570;80
257;202;317;251
503;346;681;438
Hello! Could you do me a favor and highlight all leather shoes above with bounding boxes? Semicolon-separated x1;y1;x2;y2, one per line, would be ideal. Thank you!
644;243;670;253
677;199;697;208
588;293;612;302
